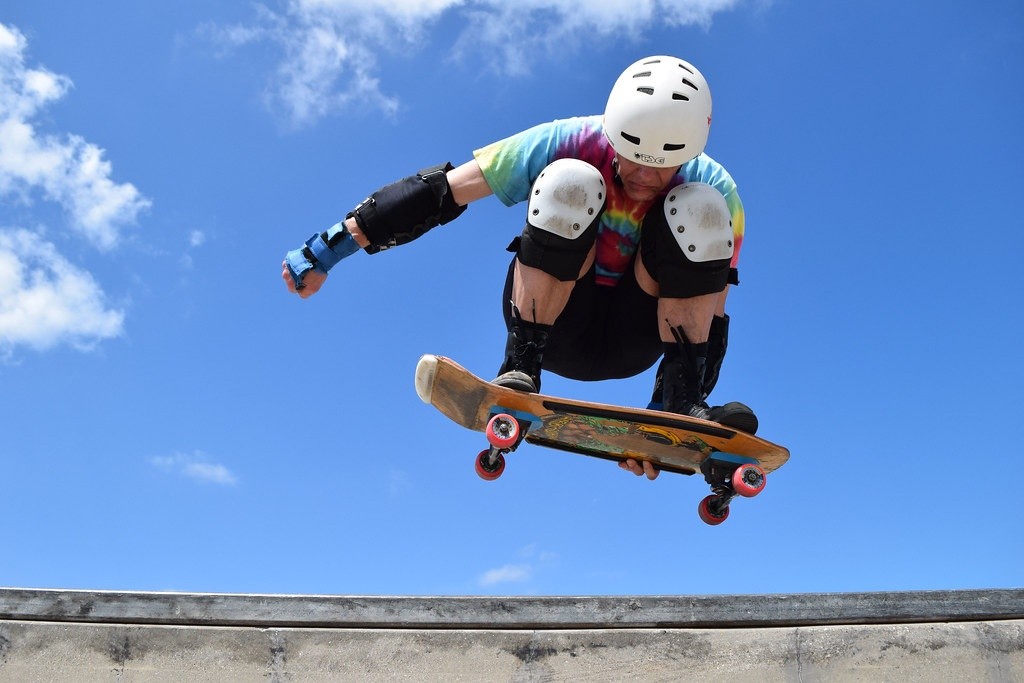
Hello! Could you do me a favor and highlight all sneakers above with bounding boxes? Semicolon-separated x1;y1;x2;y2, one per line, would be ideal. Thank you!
647;340;758;436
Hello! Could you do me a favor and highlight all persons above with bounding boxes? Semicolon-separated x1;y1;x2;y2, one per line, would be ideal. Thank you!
281;56;758;480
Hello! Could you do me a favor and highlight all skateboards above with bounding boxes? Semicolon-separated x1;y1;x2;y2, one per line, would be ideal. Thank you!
412;352;792;527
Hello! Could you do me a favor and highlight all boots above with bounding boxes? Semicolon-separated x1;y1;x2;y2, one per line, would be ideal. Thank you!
491;317;552;393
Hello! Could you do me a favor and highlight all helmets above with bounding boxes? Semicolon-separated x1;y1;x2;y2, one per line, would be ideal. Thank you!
601;54;715;169
